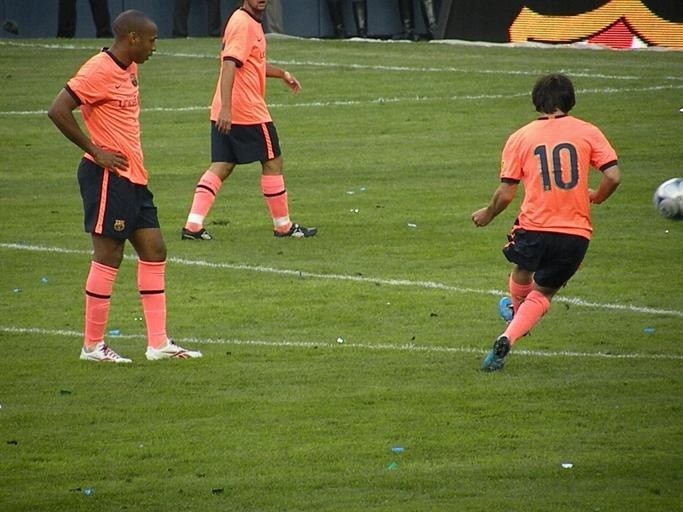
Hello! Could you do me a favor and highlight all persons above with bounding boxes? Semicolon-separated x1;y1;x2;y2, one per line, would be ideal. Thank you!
47;10;203;363
471;75;621;372
181;1;318;240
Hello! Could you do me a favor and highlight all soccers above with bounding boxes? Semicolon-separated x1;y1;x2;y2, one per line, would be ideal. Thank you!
653;177;683;221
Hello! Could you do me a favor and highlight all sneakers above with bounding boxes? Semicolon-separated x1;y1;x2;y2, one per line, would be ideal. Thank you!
272;222;318;237
78;340;133;364
484;335;509;370
500;296;531;338
145;339;202;361
181;225;213;240
